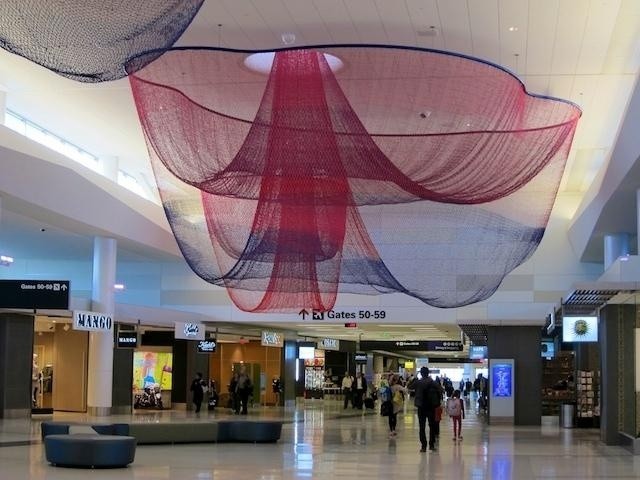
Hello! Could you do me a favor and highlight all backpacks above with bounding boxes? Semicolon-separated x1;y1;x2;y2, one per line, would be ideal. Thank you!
447;397;461;419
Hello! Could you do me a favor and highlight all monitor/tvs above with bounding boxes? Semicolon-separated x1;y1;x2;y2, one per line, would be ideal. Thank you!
538;340;556;361
561;314;599;343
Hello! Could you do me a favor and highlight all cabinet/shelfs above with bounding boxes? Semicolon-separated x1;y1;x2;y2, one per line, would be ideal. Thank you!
322;388;341;401
541;399;577;426
305;365;325;397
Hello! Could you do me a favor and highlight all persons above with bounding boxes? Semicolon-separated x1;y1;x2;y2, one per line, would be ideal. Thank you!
383;374;416;437
228;371;241;415
235;366;251;415
341;372;356;409
352;372;367;410
188;372;204;413
447;390;466;441
387;369;488;398
413;367;442;453
434;403;444;440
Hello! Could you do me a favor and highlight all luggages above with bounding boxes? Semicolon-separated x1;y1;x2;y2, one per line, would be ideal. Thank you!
364;395;375;410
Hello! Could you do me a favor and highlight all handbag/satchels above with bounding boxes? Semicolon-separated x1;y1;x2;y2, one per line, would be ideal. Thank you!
380;400;394;417
393;384;402;407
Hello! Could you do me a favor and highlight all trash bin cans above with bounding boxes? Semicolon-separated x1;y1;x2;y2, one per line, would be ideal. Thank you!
560;404;575;428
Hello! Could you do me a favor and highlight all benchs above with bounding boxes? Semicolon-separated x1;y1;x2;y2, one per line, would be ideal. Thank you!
40;419;283;469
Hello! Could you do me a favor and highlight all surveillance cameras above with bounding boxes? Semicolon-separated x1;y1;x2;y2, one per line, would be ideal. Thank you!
281;34;295;45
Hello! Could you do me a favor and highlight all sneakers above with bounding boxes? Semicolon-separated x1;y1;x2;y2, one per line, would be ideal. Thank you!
451;436;464;441
388;431;398;439
419;446;438;451
